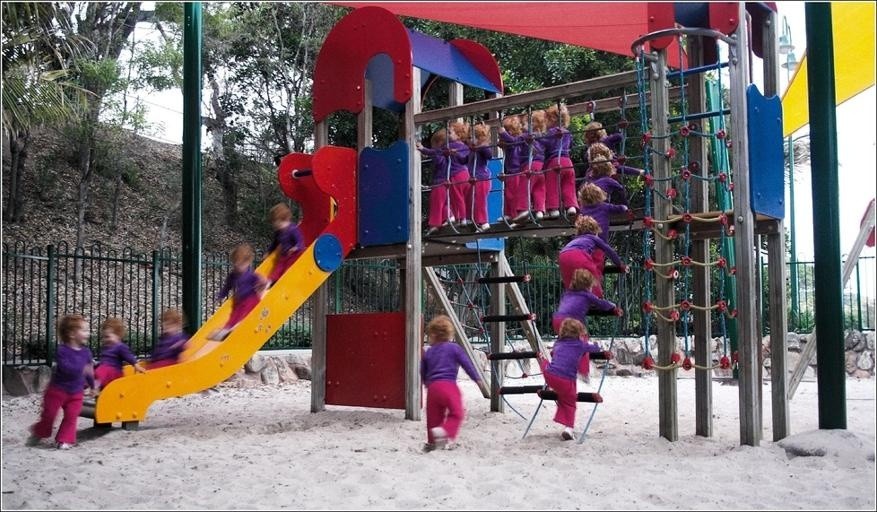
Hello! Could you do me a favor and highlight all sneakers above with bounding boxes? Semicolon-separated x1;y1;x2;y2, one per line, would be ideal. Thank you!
562;427;576;441
55;441;71;450
25;435;41;447
426;207;577;239
430;427;448;450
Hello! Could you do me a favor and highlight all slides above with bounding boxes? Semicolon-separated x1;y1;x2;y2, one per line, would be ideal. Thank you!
93;146;359;424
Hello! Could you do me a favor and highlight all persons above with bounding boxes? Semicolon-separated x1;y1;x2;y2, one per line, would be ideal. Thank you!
541;317;600;441
92;319;147;401
263;203;306;292
419;314;482;453
148;309;190;371
418;102;643;234
578;184;629;279
552;269;616;381
215;244;265;340
560;214;626;300
27;315;96;449
